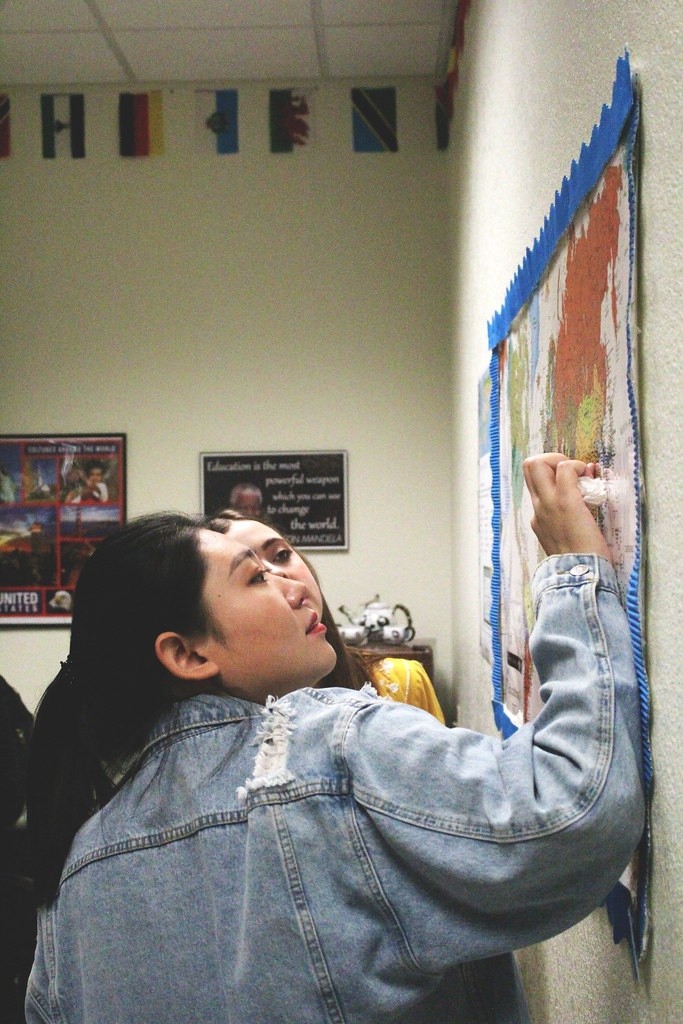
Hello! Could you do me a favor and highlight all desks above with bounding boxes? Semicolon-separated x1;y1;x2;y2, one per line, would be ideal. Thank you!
350;644;434;682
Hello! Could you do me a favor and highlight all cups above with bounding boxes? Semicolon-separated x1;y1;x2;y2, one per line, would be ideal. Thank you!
335;624;372;647
383;625;415;646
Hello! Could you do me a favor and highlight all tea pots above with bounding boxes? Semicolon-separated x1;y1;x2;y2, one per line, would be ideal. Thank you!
338;594;412;642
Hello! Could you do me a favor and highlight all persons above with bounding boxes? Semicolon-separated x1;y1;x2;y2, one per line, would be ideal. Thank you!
63;461;108;503
0;450;646;1023
232;484;263;519
